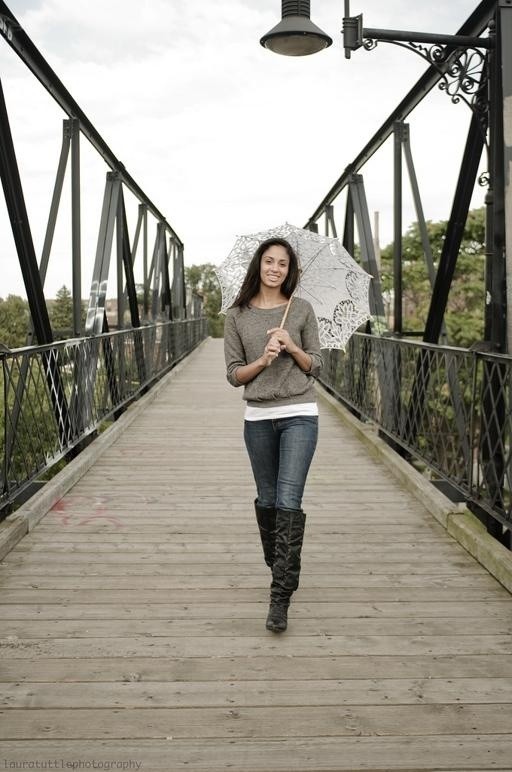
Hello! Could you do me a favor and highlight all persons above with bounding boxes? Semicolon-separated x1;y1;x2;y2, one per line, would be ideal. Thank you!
224;238;325;633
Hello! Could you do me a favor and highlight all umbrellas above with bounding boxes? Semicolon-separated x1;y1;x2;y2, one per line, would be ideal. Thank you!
210;221;374;355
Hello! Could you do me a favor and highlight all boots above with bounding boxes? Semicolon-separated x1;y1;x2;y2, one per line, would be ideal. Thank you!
265;510;306;633
254;498;300;591
